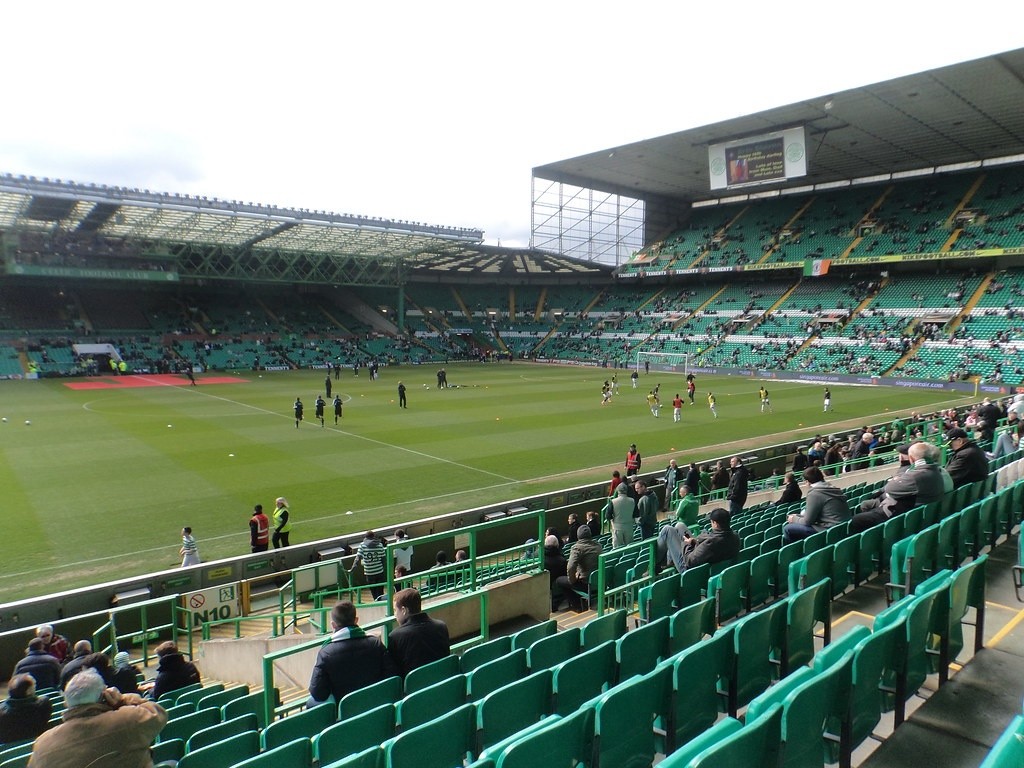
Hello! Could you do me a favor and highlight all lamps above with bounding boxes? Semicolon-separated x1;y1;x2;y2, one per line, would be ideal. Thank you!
825;98;835;110
608;151;614;158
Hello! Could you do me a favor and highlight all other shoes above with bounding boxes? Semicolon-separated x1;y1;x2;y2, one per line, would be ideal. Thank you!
986;452;996;460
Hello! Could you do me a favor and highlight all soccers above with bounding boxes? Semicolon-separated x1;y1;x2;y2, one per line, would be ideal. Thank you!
660;404;663;408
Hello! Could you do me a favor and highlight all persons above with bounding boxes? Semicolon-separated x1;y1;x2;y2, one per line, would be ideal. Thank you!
600;380;612;405
631;370;638;388
249;505;269;553
708;392;719;418
645;359;650;374
437;368;448;388
398;381;408;408
759;386;773;412
822;387;833;413
652;384;663;408
686;373;697;406
325;375;332;398
611;372;619;396
673;393;684;423
179;527;200;567
647;391;658;418
0;182;1024;386
271;497;292;549
0;386;1024;768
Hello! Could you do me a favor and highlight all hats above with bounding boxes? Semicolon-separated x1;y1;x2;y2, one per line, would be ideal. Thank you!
112;652;130;666
576;525;592;537
630;443;636;448
710;508;731;523
629;475;638;482
24;639;45;652
276;497;290;508
981;397;994;406
895;444;913;455
615;483;628;493
153;641;179;656
944;428;963;446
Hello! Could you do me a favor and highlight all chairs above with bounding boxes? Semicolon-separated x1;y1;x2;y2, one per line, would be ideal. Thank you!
0;430;1024;768
